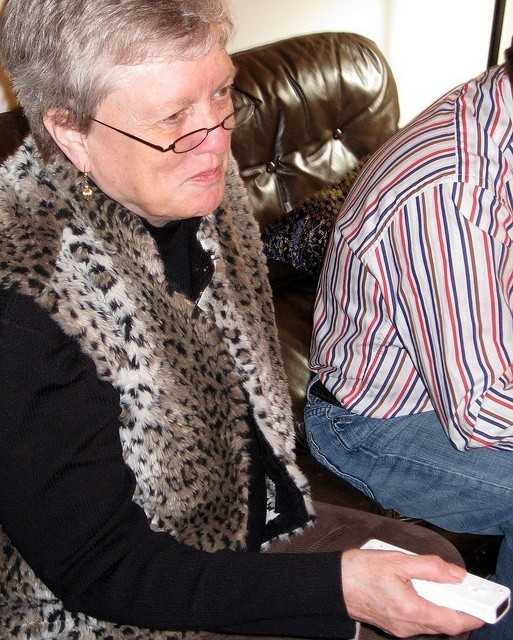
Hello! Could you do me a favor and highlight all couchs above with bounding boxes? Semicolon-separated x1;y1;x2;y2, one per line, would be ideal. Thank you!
0;30;504;577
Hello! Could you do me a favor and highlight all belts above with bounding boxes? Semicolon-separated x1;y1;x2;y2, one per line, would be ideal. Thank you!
308;371;343;405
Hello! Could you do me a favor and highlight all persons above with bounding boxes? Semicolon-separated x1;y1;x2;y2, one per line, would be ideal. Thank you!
303;35;512;640
0;0;485;640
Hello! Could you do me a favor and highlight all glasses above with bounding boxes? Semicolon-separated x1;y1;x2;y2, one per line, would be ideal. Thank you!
68;84;262;154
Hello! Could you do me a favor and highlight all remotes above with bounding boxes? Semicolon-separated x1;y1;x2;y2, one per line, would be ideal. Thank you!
358;537;512;627
